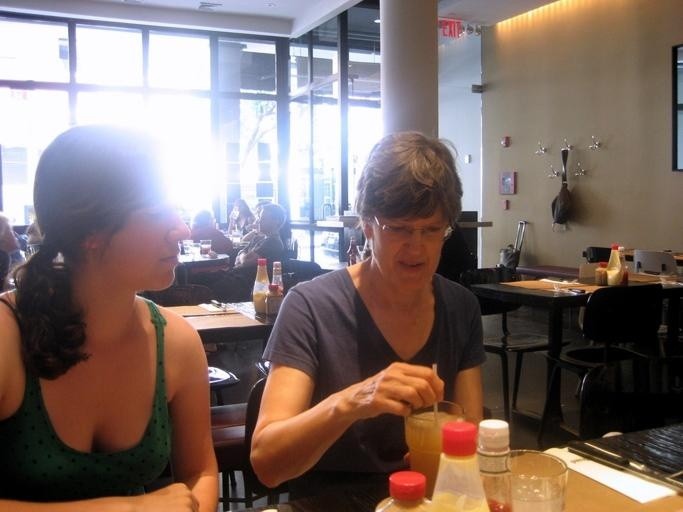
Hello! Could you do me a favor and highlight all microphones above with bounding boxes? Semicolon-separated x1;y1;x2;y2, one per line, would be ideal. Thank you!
204;366;238;405
208;365;241;511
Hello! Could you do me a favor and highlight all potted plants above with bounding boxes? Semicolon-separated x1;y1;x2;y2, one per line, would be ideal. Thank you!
498;170;515;194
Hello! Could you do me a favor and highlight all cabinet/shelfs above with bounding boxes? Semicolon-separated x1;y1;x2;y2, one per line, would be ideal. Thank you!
346;238;360;266
375;419;513;512
253;259;284;315
595;243;628;286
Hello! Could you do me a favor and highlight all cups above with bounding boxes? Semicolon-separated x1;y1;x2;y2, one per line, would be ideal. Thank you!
372;214;450;242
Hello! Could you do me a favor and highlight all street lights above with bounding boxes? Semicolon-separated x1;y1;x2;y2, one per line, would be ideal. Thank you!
499;243;517;270
550;184;573;223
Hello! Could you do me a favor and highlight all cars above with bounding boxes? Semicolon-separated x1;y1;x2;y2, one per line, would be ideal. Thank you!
403;400;462;498
343;210;352;216
183;240;194;256
232;230;241;247
233;211;239;220
200;240;211;257
506;449;568;511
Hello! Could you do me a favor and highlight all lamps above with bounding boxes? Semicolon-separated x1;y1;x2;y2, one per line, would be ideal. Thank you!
458;267;572;420
166;284;214;306
537;283;663;443
244;377;279;508
281;258;321;296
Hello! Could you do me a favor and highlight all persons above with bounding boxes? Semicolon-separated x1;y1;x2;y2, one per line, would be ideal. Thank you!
1;200;287;293
0;126;220;512
250;131;488;512
